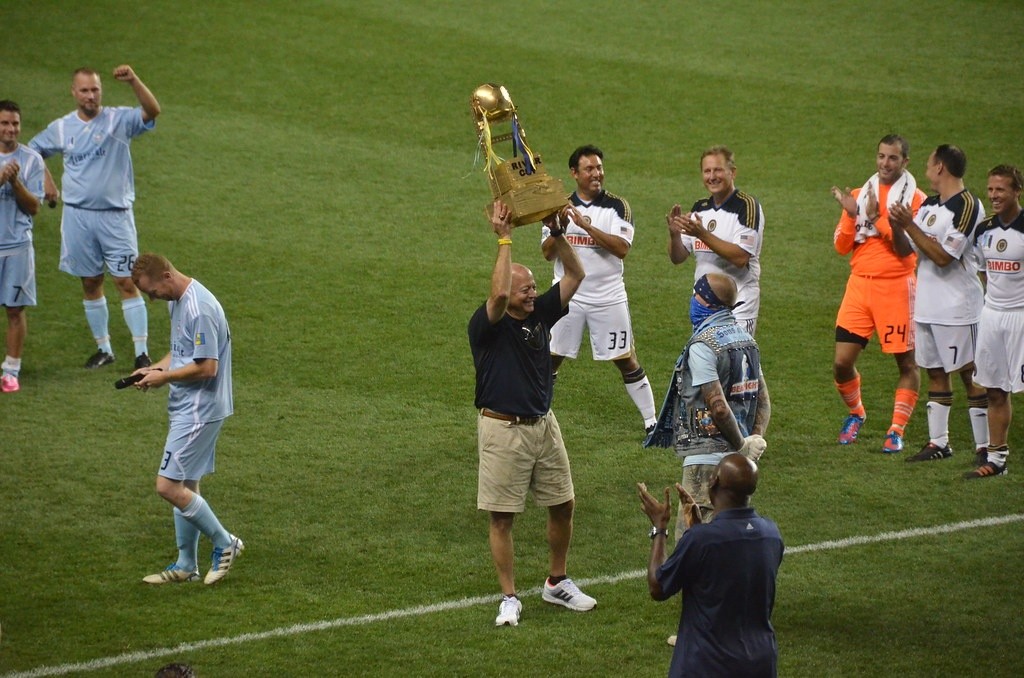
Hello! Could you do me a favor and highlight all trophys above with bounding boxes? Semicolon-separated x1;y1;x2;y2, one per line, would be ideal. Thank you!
470;83;569;229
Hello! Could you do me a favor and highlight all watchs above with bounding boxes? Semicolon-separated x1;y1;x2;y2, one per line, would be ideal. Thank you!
650;527;669;539
551;226;566;237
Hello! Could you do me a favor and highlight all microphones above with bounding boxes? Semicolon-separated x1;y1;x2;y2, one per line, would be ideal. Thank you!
115;369;163;389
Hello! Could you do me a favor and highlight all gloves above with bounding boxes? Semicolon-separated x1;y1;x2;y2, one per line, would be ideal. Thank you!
737;434;762;457
748;438;767;461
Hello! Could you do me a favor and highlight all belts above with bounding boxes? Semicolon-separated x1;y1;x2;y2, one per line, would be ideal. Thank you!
479;407;547;425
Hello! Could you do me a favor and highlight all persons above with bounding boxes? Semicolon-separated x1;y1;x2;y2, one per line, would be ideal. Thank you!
0;100;45;393
130;254;246;585
644;274;770;646
542;144;657;436
960;165;1024;479
666;144;765;338
468;202;597;625
832;134;928;453
637;453;784;678
26;65;161;369
887;144;990;465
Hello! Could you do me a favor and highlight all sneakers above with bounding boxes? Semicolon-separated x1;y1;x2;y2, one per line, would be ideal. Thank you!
204;533;245;585
0;367;20;393
135;352;152;369
143;562;200;583
966;462;1008;481
838;411;866;445
976;447;988;466
542;576;597;612
496;595;522;627
85;348;116;370
882;430;904;453
905;442;953;462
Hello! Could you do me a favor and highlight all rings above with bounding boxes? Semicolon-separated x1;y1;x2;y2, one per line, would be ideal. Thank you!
499;215;505;220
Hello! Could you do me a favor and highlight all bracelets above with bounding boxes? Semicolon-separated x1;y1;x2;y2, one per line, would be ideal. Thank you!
498;239;513;245
871;216;879;223
